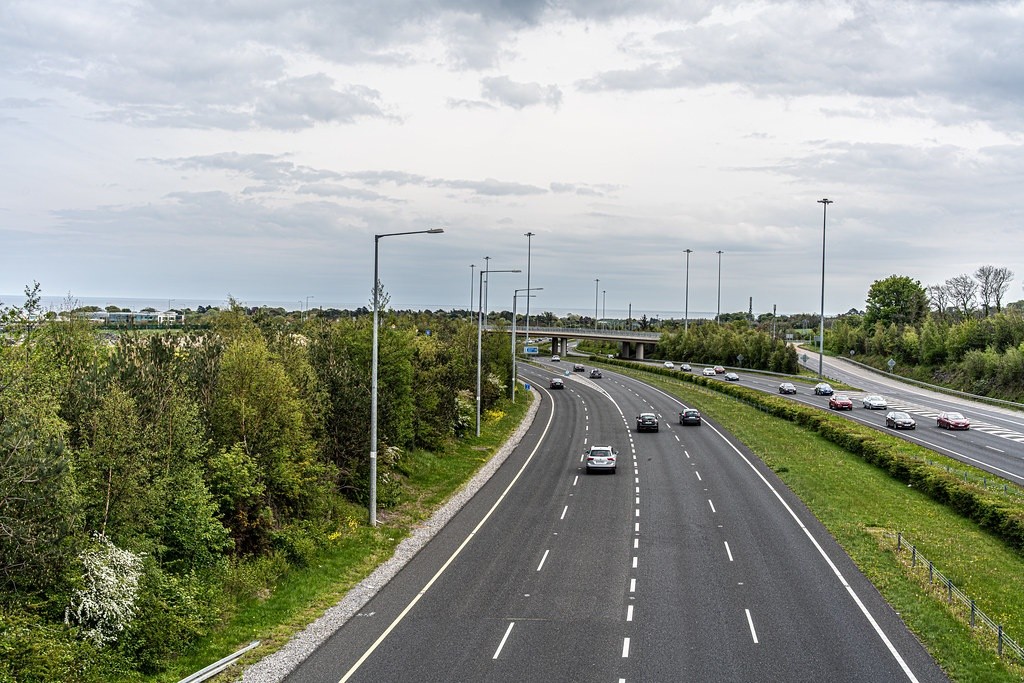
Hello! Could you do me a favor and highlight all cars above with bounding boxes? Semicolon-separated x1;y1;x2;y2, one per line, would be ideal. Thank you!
679;408;702;427
528;339;543;344
863;395;888;410
885;412;916;430
550;355;603;389
636;413;659;433
937;412;970;431
779;383;834;396
829;394;853;411
585;445;620;474
663;361;739;381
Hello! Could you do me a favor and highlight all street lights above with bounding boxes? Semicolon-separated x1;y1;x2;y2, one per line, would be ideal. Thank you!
524;232;535;328
513;288;543;403
817;198;833;379
468;256;492;326
716;251;724;325
369;228;444;527
594;279;607;330
683;249;693;333
478;270;522;438
